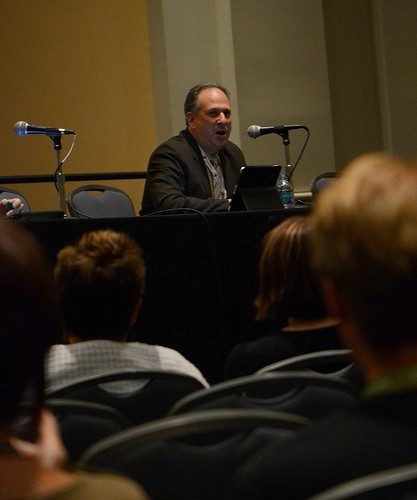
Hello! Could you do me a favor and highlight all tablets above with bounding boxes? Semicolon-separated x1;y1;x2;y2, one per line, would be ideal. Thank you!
227;164;281;211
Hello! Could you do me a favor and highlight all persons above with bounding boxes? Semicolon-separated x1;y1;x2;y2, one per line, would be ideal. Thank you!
140;83;252;212
0;154;417;500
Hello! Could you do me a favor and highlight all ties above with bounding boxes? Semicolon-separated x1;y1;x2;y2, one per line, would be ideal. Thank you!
209;157;228;201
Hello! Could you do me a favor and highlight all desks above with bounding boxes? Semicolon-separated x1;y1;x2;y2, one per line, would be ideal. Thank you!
0;208;310;417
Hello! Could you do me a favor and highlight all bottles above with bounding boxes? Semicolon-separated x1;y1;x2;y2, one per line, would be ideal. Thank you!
277;175;294;209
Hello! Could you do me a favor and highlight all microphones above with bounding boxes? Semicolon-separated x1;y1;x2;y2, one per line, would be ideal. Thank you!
14;120;76;137
246;124;306;138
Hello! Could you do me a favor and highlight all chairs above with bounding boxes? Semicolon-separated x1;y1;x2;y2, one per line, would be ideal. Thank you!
46;351;417;500
68;184;137;217
0;188;30;213
